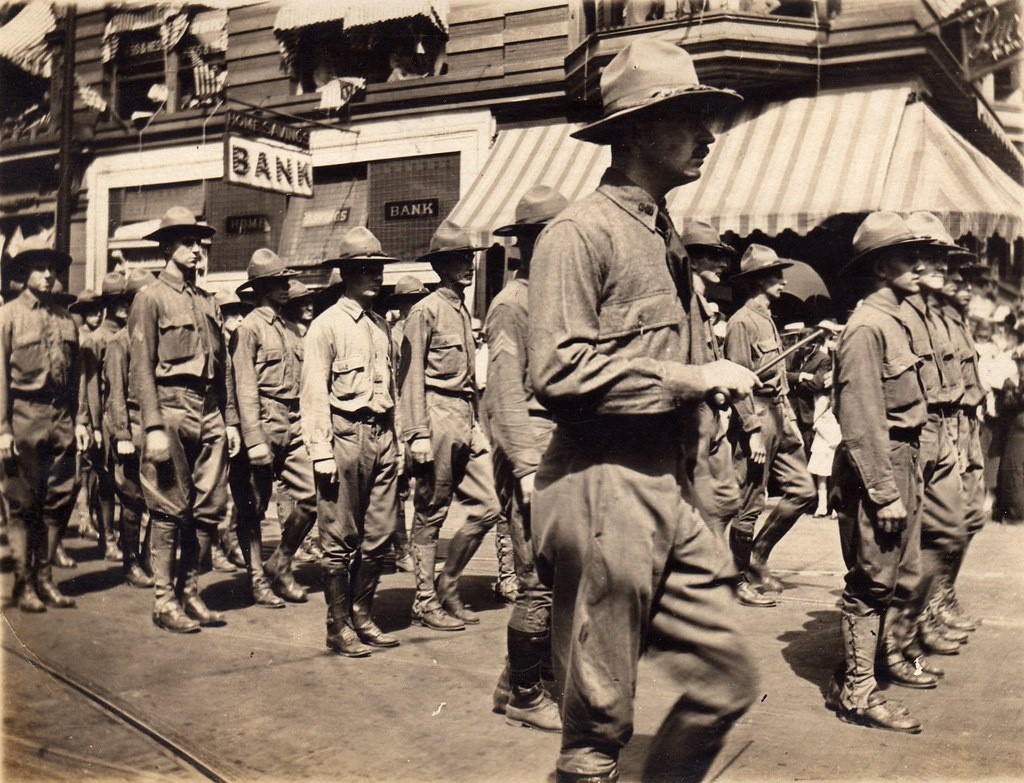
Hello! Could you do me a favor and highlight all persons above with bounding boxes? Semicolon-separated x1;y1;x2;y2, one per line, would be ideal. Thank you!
0;235;157;613
826;211;937;734
129;206;317;635
299;220;502;658
876;212;1024;688
531;37;763;783
479;185;843;733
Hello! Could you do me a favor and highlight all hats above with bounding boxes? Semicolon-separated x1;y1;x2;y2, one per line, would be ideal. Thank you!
569;41;744;146
725;243;794;280
681;220;737;252
845;211;1024;315
142;207;218;240
206;184;578;313
780;322;814;336
6;235;158;311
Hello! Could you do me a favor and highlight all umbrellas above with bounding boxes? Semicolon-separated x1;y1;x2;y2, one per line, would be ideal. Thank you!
775;257;831;301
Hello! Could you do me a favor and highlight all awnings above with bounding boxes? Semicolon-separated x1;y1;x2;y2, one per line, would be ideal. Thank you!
874;99;1024;241
429;76;929;238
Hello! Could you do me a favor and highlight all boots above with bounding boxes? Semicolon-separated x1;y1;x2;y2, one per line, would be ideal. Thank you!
640;695;747;783
750;492;814;592
505;624;563;732
493;659;510;710
556;767;620;783
823;549;981;733
728;525;776;607
0;490;487;657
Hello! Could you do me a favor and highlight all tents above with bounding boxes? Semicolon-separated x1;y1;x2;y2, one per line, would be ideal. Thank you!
273;0;450;78
